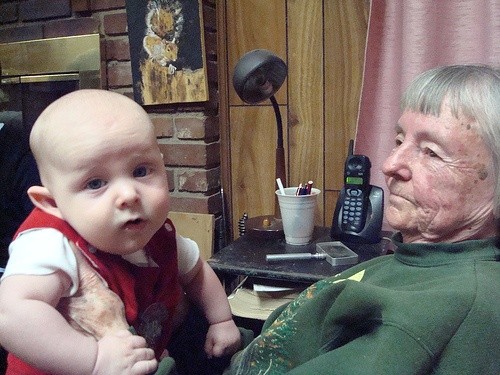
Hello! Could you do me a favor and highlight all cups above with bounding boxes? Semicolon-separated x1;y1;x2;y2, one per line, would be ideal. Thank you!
274;187;321;245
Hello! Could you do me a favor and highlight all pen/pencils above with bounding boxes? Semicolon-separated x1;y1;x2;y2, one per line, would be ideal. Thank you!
276;178;285;194
295;180;313;196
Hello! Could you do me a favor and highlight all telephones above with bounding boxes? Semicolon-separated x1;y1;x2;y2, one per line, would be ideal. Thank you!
331;139;384;244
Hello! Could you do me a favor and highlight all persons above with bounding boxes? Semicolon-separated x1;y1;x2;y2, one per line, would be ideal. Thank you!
0;89;238;375
55;63;499;375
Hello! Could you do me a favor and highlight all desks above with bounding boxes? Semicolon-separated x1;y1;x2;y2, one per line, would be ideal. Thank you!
206;225;403;334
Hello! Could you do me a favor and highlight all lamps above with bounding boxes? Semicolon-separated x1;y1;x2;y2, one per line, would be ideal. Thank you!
232;49;288;238
